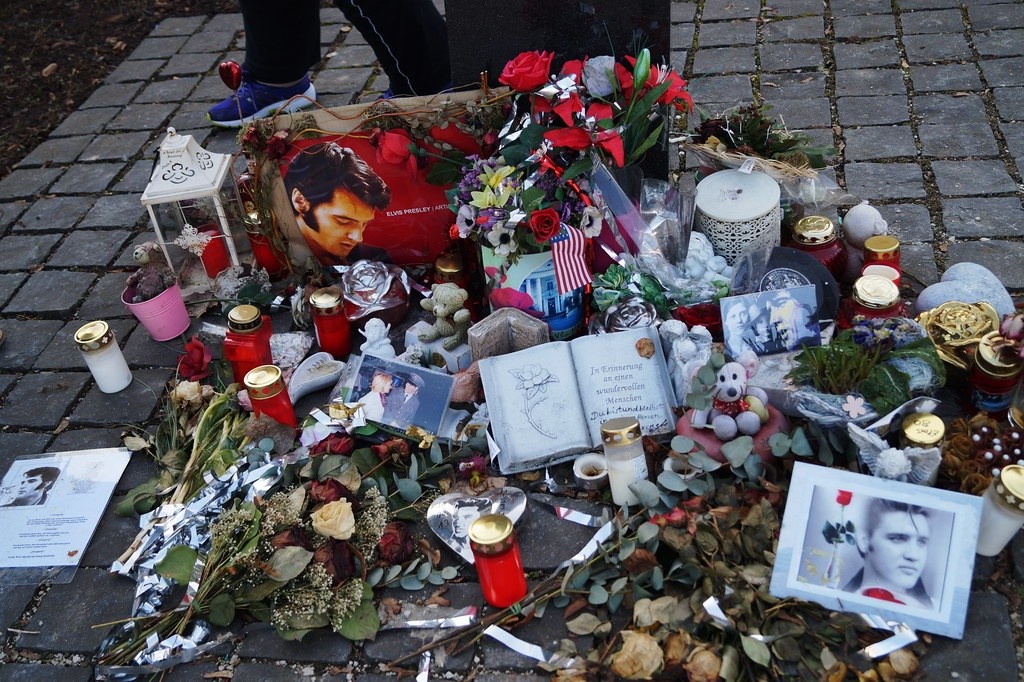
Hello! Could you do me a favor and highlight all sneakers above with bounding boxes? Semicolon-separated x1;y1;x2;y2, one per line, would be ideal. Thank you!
375;86;396;101
205;63;317;127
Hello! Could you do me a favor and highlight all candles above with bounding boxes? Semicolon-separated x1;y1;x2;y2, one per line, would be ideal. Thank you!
74;319;132;393
978;465;1024;556
242;365;297;428
310;287;352;359
432;253;479;322
243;209;286;271
466;512;531;609
194;222;231;279
791;216;909;330
970;334;1024;396
601;418;649;506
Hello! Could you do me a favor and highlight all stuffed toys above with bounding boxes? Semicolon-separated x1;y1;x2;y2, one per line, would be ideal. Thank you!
126;242;176;304
418;283;471;349
690;351;768;439
842;203;887;275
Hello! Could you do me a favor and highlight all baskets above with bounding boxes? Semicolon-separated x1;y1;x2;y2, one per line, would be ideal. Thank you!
691;138;813;181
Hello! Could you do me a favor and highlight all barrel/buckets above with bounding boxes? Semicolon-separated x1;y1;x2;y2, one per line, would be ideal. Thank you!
120;271;191;342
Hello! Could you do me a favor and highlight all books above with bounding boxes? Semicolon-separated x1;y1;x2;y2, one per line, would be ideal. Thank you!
467;307;549;365
479;327;681;475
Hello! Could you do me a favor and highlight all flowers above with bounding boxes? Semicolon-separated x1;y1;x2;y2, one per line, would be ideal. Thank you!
692;94;836;170
90;339;930;682
445;43;694;265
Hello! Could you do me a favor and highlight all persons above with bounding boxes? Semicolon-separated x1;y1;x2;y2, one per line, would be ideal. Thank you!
449;499;492;564
207;0;471;126
724;287;820;357
359;318;394;359
358;372;426;430
875;448;912;481
673;233;733;295
252;144;393;282
842;500;935;609
0;466;61;506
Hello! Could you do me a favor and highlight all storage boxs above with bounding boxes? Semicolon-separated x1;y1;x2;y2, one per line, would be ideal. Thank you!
404;322;471;375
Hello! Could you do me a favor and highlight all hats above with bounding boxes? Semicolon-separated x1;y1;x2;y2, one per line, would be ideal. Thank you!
743;303;770;331
405;372;425;389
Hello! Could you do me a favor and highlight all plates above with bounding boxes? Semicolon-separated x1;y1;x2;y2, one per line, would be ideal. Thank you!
733;245;841;334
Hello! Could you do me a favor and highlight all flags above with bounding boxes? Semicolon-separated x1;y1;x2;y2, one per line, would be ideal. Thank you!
551;223;595;295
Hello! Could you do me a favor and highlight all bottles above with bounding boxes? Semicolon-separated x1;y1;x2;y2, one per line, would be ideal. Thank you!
74;320;133;394
600;417;651;507
243;365;301;438
468;513;528;609
896;413;946;487
784;215;909;330
431;257;466;304
308;288;351;358
976;464;1024;557
223;304;274;390
967;331;1024;414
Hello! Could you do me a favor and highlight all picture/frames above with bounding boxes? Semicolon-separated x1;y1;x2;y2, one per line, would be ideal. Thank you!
770;459;984;641
243;84;515;268
